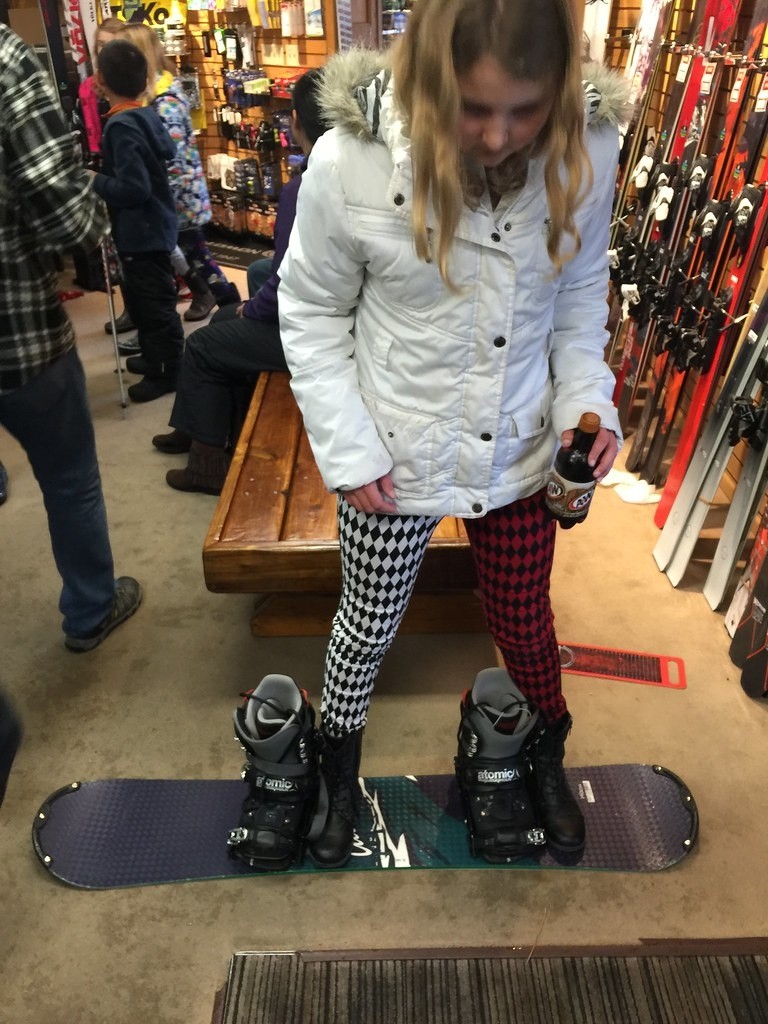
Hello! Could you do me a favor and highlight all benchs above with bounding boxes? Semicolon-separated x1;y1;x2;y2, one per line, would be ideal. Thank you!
203;369;489;637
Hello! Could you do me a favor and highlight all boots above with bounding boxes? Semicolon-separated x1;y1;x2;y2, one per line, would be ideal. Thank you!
184;273;217;321
152;426;192;453
307;720;365;868
166;440;227;497
529;711;587;853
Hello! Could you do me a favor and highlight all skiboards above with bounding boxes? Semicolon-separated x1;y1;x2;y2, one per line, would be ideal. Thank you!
609;0;768;701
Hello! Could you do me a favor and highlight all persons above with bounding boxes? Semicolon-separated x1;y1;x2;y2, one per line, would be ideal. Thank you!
275;0;636;870
0;19;334;651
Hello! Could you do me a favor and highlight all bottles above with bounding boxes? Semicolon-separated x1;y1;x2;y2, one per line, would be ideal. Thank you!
544;413;601;529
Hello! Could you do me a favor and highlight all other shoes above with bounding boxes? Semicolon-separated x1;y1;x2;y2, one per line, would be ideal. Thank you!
118;333;141;354
0;460;8;505
104;308;137;334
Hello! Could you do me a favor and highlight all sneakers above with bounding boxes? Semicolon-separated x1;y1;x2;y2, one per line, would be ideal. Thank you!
176;274;193;300
455;667;539;863
226;674;317;868
64;577;141;651
128;375;175;402
126;356;155;375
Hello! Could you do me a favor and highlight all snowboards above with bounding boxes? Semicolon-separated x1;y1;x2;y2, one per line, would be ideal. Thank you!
31;760;701;891
